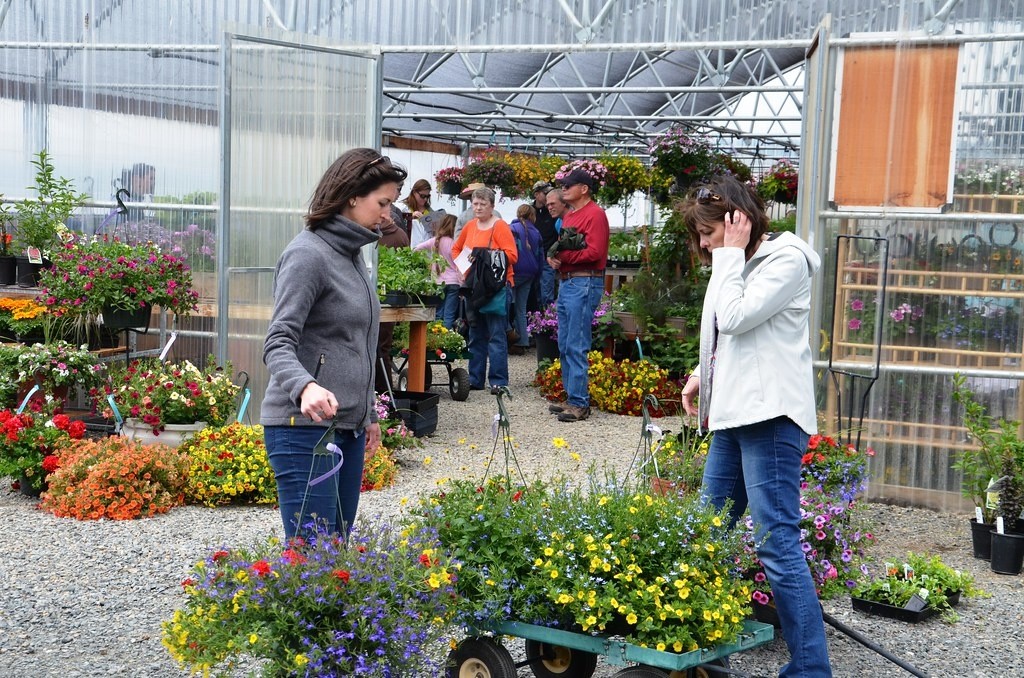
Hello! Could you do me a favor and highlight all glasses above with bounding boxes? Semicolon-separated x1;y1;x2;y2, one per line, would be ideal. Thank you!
416;191;431;198
685;187;722;204
561;184;573;190
366;155;392;167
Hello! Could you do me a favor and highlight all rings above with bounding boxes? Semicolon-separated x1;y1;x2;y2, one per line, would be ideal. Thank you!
317;409;324;417
733;221;740;224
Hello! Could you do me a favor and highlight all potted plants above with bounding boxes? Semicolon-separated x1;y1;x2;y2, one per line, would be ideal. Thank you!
989;449;1024;575
0;145;92;289
950;449;997;562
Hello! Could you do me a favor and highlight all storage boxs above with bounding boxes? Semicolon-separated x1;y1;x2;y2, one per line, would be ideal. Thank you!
389;390;440;439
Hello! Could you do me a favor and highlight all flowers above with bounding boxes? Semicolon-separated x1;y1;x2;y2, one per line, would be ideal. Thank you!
434;130;799;220
0;229;16;256
956;164;1024;198
0;222;1024;678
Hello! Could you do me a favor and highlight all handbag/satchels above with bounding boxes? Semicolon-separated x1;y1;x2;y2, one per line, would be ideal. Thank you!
474;276;507;316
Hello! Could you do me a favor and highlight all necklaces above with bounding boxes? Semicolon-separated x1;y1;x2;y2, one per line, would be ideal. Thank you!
471;216;494;243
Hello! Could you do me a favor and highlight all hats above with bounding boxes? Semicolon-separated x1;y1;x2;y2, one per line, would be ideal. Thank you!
532;183;554;194
555;170;593;190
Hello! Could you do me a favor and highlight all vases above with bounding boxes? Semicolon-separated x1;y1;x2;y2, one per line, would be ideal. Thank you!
0;184;1010;634
0;256;16;285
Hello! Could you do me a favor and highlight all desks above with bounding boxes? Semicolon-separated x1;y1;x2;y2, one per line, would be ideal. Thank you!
151;302;436;392
603;332;686;360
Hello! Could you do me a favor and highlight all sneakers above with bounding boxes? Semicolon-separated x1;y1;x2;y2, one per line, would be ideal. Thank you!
549;401;591;415
558;406;589;421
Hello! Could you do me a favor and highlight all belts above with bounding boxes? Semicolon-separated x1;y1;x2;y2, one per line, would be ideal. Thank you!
561;270;603;280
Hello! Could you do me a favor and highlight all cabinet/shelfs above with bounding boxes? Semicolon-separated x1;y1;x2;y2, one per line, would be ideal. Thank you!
823;195;1024;511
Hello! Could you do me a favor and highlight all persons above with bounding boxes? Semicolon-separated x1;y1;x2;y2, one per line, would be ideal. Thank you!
681;175;834;678
262;149;409;555
377;170;610;422
113;164;156;228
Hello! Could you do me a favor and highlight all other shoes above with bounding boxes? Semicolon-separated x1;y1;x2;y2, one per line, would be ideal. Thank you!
491;385;500;394
508;331;521;347
509;346;526;355
